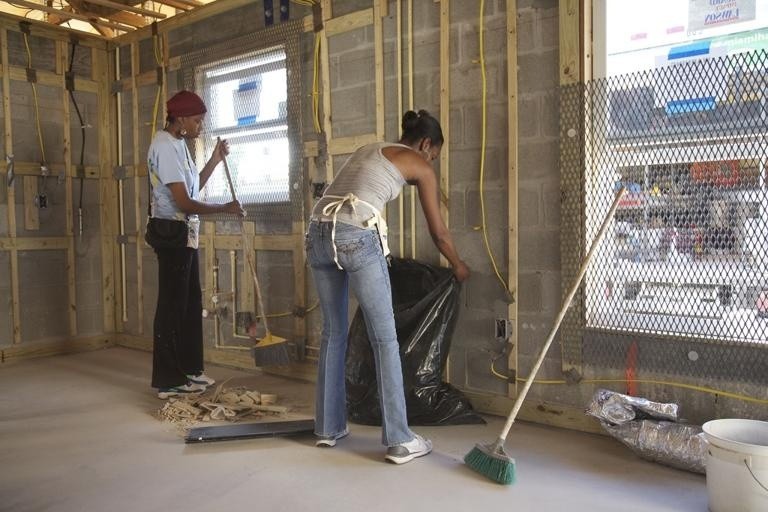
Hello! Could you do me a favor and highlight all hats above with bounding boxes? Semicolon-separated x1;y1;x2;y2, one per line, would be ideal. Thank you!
166;90;208;119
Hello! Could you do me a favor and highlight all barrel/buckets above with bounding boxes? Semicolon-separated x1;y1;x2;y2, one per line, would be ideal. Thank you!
702;418;768;512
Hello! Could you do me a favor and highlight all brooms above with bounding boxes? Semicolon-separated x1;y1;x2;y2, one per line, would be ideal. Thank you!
218;136;292;368
464;186;627;486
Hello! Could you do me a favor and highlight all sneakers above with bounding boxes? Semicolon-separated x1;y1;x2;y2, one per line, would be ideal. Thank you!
157;378;207;400
314;422;350;447
383;432;434;465
184;371;216;387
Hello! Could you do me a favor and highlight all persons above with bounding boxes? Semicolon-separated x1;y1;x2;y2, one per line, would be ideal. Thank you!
144;90;249;400
303;109;471;465
